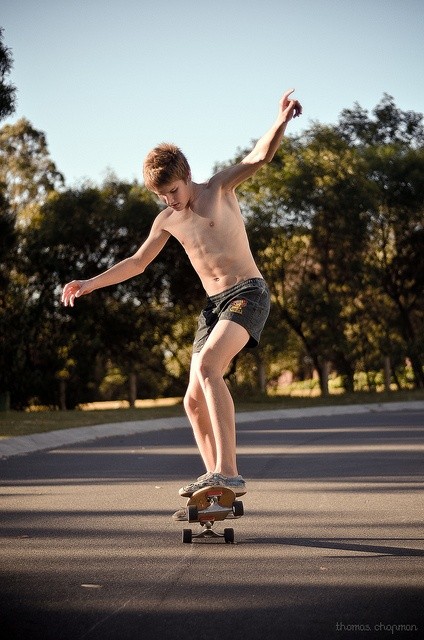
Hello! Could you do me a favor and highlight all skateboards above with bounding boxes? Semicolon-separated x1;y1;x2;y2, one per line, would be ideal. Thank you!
182;486;244;543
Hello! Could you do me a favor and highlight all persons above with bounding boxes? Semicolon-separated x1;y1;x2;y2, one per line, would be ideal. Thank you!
62;88;302;521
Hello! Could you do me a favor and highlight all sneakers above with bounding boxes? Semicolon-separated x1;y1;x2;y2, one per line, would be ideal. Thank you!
179;472;248;497
172;507;243;521
